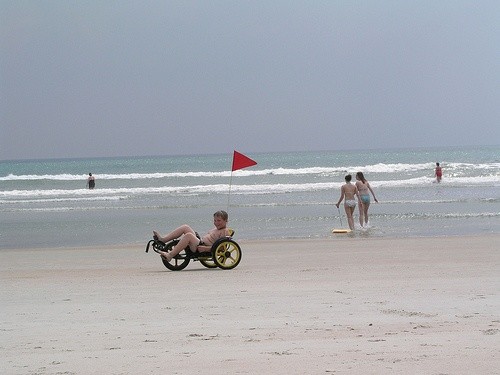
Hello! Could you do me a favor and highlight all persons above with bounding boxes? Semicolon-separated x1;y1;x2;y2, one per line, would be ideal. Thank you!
88;173;95;189
153;210;228;261
336;172;378;231
435;163;442;183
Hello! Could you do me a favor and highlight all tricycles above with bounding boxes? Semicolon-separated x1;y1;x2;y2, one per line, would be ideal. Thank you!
144;227;242;271
